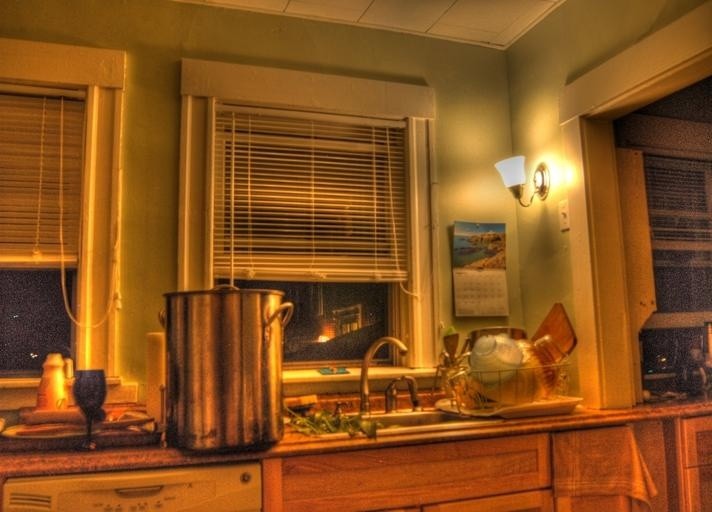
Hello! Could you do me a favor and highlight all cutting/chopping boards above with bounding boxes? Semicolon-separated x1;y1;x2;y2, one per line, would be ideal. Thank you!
531;302;578;364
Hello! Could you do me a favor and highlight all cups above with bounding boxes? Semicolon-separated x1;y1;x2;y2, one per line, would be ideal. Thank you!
446;366;485;414
72;370;107;453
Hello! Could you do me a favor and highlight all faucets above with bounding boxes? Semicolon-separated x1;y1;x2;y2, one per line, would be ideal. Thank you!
359;336;409;415
384;375;402;413
401;375;423;410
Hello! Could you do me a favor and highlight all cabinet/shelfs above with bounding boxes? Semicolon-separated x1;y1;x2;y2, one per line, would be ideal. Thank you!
680;411;712;512
268;424;553;511
1;459;263;511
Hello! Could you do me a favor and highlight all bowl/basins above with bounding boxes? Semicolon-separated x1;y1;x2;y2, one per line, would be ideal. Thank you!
470;332;523;390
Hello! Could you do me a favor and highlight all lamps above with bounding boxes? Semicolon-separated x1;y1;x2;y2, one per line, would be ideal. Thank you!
494;155;550;208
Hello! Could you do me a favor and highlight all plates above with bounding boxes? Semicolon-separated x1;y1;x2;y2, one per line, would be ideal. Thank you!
435;396;583;418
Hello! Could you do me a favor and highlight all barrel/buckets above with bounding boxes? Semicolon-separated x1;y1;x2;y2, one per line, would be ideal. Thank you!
158;284;293;453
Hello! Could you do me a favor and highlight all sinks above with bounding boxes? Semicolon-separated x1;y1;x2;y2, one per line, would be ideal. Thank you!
313;409;505;440
